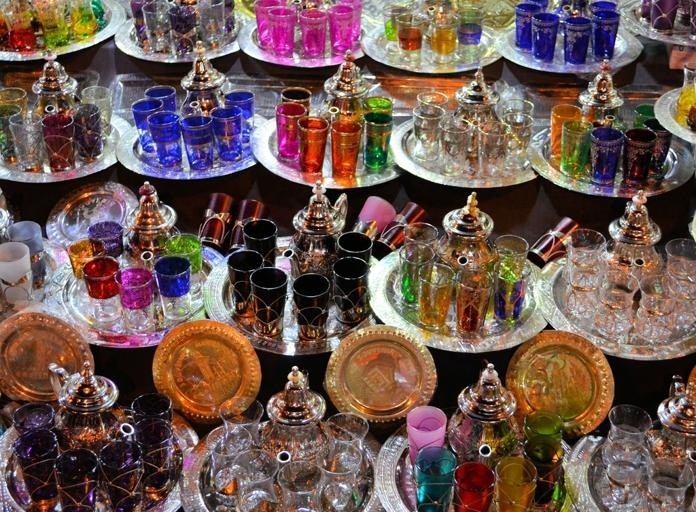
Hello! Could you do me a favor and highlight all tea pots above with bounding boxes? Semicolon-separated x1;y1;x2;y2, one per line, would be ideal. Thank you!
552;0;593;32
117;180;174;275
439;64;510;164
578;58;629;137
46;360;135;458
319;49;373;122
600;190;662;304
31;48;83;119
291;0;320;16
422;1;451;27
445;358;521;470
646;374;696;502
425;193;501;305
281;179;349;304
177;40;233;120
259;364;330;490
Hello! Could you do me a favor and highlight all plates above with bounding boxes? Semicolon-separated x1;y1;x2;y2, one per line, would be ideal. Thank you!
45;180;142;254
525;119;694;198
0;406;199;512
0;312;95;408
0;239;73;323
44;240;226;349
373;421;588;512
388;118;539;189
114;16;253;64
152;319;262;427
504;328;616;441
249;112;406;191
360;20;503;75
496;25;645;76
532;250;696;362
115;110;270;181
367;244;548;354
201;235;380;357
1;115;132;184
653;85;696;146
323;324;439;426
178;421;382;512
619;4;696;48
564;433;695;511
0;0;128;63
237;14;375;68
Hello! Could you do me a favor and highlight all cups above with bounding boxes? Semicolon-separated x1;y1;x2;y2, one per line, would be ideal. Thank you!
453;461;496;511
298;9;328;60
8;111;45;170
0;105;28;169
527;216;582;269
139;0;177;54
395;11;426;62
209;103;244;164
132;417;174;503
566;228;607;292
323;411;370;484
602;403;654;470
412;103;446;160
334;231;373;268
438;116;473;177
249;268;289;337
493;259;532;329
416;90;450;109
631;102;654;127
280;85;313;116
71;103;104;165
639;1;653;23
592;270;640;345
292;273;330;342
30;1;70;45
223;88;255;159
340;0;362;43
295;115;330;173
41;112;77;173
375;201;428;252
405;405;447;467
456;7;486;67
218;395;263;449
205;424;253;507
414;444;458;511
644;458;694;511
211;1;236;34
231;449;280;511
1;0;37;53
229;198;265;249
362;111;394;173
351;195;396;242
530;12;561;65
522;409;564;462
276;460;325;512
589;126;624;187
316;441;363;512
130;96;165;158
163;232;204;302
676;62;696;128
144;83;178;113
332;256;370;325
665;237;696;312
178;114;216;172
330;120;362;180
114;266;156;337
99;439;146;511
524;435;565;508
643;118;672;178
131;392;173;425
225;248;264;326
81;85;115;148
146;112;183;166
86;220;125;271
425;12;462;65
81;254;122;329
52;447;99;511
417;262;457;331
653;1;678;32
604;438;651;511
242;218;278;267
563;15;594;66
514;2;543;55
0;87;30;119
65;237;105;302
6;220;46;278
624;128;658;190
153;255;193;322
11;429;60;511
550;104;584;163
499;112;535;172
382;1;409;56
0;241;34;314
493;234;531;261
558;120;592;177
477;120;512;179
398;240;436;309
590;9;622;64
633;274;682;343
130;1;157;46
403;221;439;258
455;266;493;338
587;1;617;14
274;103;309;163
194;0;227;54
267;5;297;59
167;5;201;56
253;0;289;52
66;0;99;40
494;455;538;511
361;96;395;119
197;192;237;249
11;401;57;436
500;98;536;118
328;5;354;56
